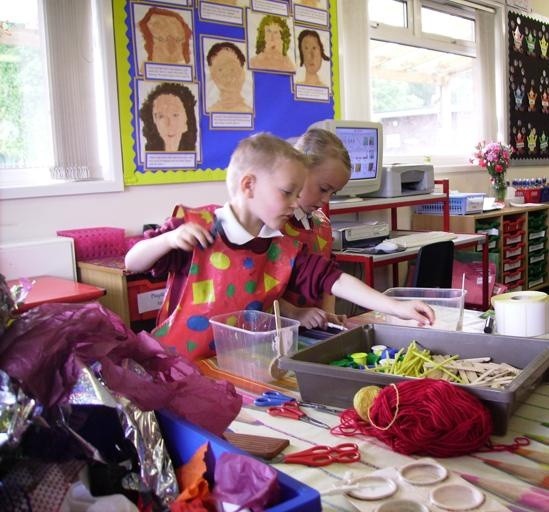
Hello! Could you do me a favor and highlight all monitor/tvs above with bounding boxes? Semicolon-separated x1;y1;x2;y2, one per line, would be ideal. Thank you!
286;118;383;203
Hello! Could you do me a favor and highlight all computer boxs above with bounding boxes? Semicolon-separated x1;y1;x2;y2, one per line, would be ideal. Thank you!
329;219;389;250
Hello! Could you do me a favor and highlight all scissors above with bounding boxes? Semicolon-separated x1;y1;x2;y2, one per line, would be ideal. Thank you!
254;390;330;429
261;442;361;467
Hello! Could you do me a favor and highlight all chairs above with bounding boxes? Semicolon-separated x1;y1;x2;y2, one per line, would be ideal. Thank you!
413;240;455;289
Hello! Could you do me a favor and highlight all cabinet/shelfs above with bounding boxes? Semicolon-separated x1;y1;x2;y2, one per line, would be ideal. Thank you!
412;201;549;294
78;262;173;342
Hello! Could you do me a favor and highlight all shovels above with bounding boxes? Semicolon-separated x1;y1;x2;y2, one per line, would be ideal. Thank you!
222;431;290;459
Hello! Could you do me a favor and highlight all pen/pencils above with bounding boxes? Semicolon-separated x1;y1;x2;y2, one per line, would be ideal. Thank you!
447;421;549;512
234;409;265;426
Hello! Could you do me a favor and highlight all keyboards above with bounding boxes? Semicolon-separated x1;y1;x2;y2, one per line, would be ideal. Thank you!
386;230;457;251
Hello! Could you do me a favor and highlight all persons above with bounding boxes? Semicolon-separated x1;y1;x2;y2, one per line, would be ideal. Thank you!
124;131;435;357
139;8;330;151
277;128;355;331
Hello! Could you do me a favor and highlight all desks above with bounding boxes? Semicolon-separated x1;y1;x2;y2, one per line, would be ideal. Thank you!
169;293;548;512
320;180;492;316
9;274;105;311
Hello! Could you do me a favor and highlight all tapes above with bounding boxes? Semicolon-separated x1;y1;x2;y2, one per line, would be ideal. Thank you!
494;290;549;338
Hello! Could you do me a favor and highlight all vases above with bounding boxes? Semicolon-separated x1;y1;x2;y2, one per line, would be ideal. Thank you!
493;181;509;203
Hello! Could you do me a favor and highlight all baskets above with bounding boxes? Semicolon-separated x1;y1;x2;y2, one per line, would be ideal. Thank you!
416;193;486;214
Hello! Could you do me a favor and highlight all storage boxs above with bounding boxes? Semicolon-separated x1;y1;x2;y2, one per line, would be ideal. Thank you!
382;284;465;334
208;310;302;388
473;209;549;293
536;186;549;202
515;182;540;203
277;319;549;439
0;234;77;285
151;414;325;512
131;277;165;324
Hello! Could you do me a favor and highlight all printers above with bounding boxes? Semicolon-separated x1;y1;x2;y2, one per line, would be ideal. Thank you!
360;164;436;199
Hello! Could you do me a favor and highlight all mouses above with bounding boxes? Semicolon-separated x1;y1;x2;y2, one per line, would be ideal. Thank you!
375;240;405;253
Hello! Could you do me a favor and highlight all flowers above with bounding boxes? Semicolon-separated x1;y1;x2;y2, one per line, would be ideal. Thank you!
469;141;515;181
